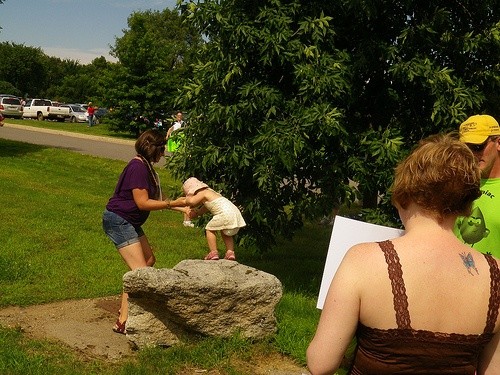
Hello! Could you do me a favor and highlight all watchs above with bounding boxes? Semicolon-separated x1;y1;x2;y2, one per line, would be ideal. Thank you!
166;200;171;210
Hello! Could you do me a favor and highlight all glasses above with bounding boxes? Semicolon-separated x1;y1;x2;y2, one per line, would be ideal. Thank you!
467;144;484;151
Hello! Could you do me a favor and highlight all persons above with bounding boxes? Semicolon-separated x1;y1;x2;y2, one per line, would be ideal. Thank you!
183;205;198;228
104;129;190;335
166;112;188;140
87;102;100;127
181;177;246;260
306;130;500;375
453;115;500;261
142;116;163;129
20;98;26;107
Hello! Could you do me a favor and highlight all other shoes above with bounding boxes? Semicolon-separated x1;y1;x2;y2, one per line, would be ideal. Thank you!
204;251;219;259
223;250;235;260
113;309;125;335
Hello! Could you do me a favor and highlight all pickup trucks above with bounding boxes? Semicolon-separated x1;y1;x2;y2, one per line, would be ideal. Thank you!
22;99;70;122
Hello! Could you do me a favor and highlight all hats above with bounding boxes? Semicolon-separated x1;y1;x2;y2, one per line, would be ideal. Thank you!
182;177;207;196
459;114;500;144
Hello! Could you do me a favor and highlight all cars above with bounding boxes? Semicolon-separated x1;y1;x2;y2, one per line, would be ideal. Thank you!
80;104;109;126
59;104;89;123
0;96;24;119
